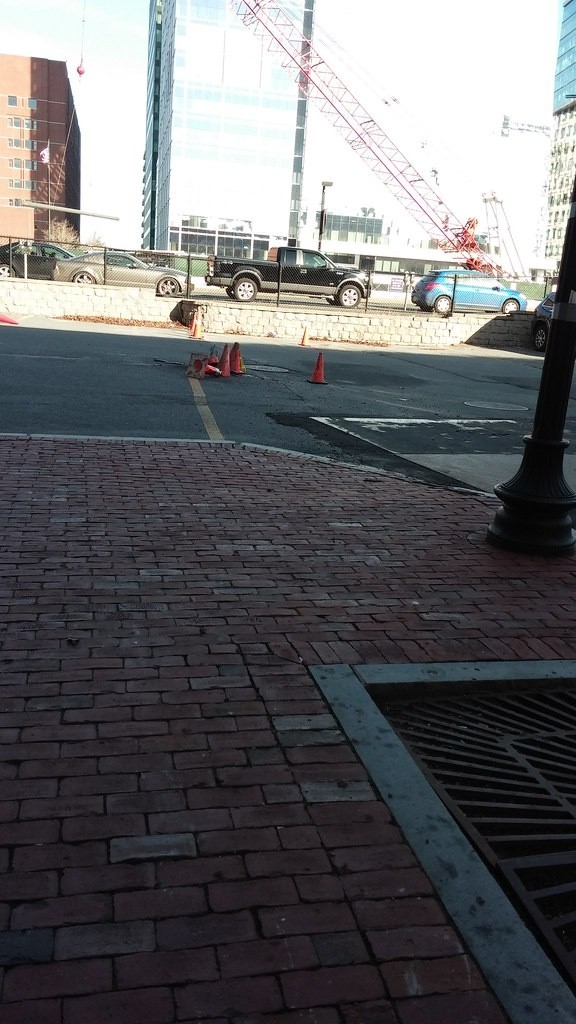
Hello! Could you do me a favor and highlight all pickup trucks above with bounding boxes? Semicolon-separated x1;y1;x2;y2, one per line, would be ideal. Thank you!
205;246;372;308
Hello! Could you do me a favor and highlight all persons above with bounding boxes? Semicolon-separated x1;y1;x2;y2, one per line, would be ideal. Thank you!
49;252;56;258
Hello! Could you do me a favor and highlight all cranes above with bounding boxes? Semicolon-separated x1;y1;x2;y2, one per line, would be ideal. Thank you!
498;113;551;138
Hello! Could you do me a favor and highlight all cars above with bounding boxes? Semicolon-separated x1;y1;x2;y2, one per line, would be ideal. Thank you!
410;269;528;314
0;242;76;279
530;292;557;351
52;250;195;297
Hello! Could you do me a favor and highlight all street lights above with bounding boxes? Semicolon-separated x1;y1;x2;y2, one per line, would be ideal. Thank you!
318;181;333;252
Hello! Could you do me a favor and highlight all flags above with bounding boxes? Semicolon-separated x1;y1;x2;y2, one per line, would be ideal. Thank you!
39;145;50;164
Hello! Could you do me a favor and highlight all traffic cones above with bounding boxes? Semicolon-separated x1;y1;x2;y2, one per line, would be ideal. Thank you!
187;352;222;379
217;343;231;377
188;306;204;340
299;326;311;346
307;353;328;384
1;313;20;325
230;342;247;375
